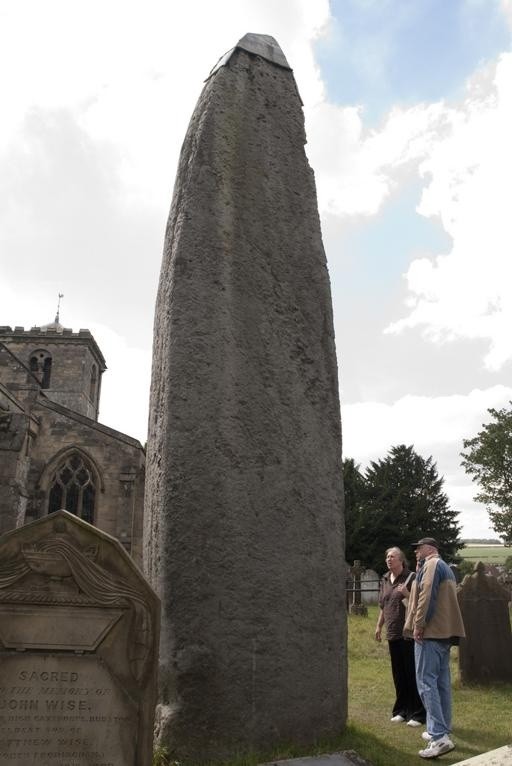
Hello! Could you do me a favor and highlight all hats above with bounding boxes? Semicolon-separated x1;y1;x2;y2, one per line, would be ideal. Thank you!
410;537;440;549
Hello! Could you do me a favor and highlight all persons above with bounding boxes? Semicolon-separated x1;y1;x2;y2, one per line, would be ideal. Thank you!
375;546;427;727
402;537;466;759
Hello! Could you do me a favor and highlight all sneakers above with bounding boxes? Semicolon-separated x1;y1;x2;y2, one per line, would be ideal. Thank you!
407;719;422;727
418;731;455;759
390;715;405;722
